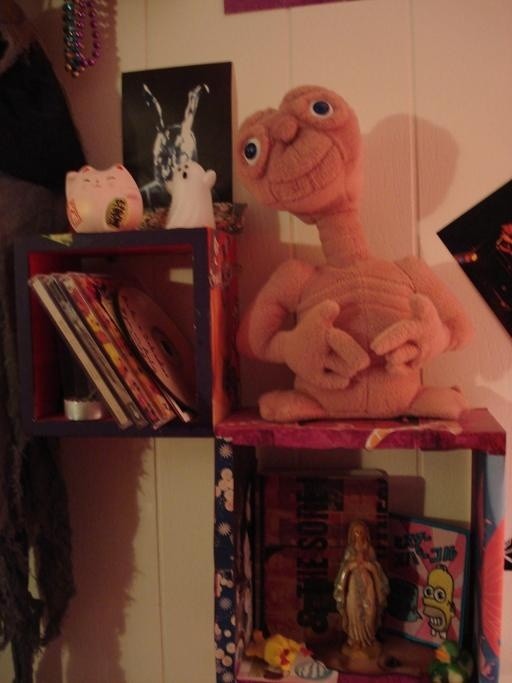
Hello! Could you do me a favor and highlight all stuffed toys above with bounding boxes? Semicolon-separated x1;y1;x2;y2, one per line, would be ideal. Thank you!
236;85;473;423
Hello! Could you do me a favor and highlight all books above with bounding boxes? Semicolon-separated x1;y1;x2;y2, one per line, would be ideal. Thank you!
31;272;197;431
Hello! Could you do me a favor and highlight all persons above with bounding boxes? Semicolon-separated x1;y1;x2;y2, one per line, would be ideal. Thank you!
334;521;390;647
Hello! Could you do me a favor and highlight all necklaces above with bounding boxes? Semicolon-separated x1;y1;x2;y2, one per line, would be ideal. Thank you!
75;1;99;68
64;1;84;77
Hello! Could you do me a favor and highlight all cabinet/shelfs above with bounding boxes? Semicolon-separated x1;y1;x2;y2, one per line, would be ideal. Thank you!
14;227;512;682
